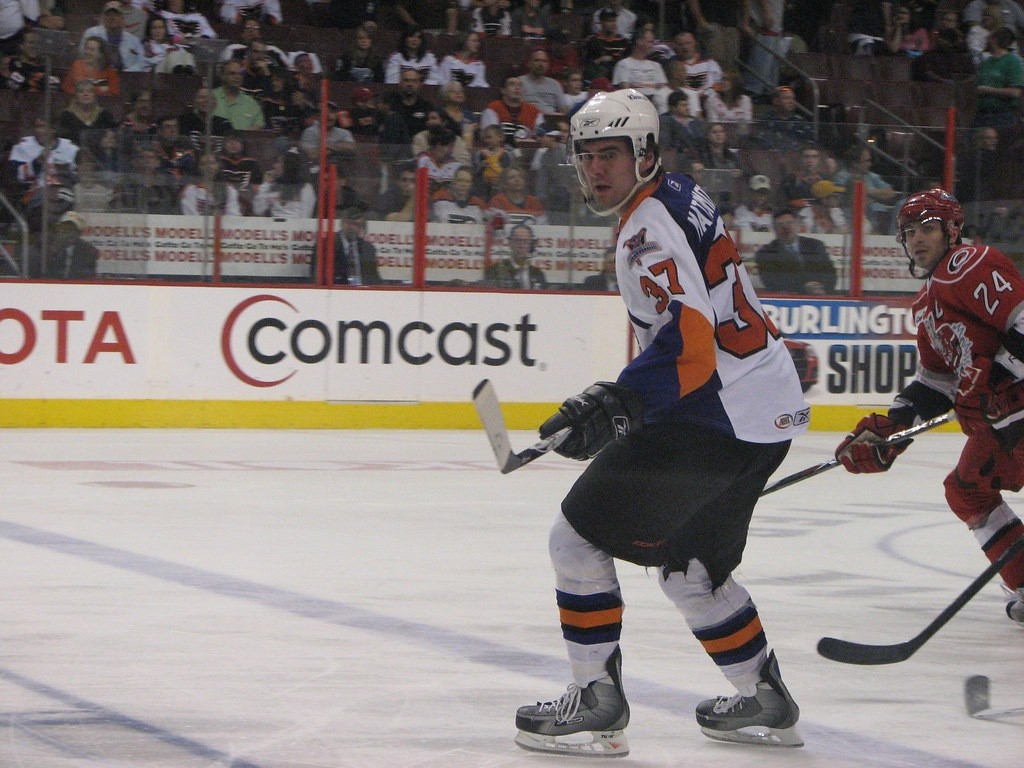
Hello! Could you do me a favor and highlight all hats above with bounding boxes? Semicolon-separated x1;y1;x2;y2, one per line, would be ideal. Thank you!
61;211;87;230
103;1;123;13
750;175;771;190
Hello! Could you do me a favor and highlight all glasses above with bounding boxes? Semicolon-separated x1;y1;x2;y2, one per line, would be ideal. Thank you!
243;26;261;29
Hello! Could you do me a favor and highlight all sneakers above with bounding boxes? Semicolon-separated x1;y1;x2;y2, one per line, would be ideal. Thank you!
514;644;631;759
696;649;803;749
1001;582;1024;623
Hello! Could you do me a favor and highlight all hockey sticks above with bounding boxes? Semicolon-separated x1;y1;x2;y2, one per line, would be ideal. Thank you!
471;377;573;474
818;532;1023;665
760;405;958;502
965;674;1023;719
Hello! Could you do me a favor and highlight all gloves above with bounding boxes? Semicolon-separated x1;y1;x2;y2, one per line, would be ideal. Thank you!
541;381;642;463
835;413;914;475
954;358;1003;437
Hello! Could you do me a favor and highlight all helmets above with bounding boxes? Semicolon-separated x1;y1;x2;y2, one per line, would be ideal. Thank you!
897;187;965;231
570;89;659;157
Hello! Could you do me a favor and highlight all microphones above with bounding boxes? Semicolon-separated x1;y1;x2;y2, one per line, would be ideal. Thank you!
520;253;535;260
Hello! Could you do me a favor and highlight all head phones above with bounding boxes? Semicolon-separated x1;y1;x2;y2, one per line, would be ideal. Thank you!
509;224;536;253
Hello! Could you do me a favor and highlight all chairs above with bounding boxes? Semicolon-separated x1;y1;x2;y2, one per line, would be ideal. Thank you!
0;0;1024;273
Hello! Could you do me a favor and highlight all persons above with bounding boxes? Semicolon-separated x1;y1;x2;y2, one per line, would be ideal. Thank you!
516;87;809;736
835;188;1024;621
0;0;1024;295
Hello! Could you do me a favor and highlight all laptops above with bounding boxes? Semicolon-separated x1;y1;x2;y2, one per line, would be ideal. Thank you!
702;169;735;192
